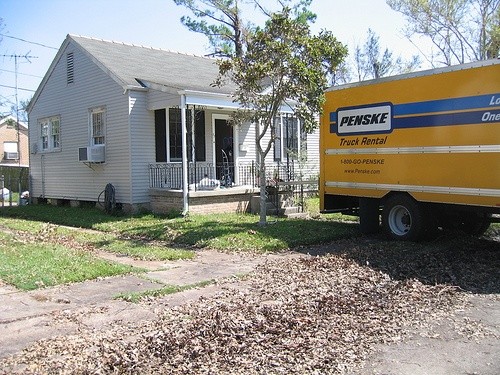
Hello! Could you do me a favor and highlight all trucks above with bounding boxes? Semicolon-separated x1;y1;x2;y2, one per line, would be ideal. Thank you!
319;56;500;242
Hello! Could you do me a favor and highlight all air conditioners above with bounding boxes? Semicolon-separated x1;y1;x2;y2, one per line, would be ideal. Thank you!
4;151;20;160
78;144;105;163
